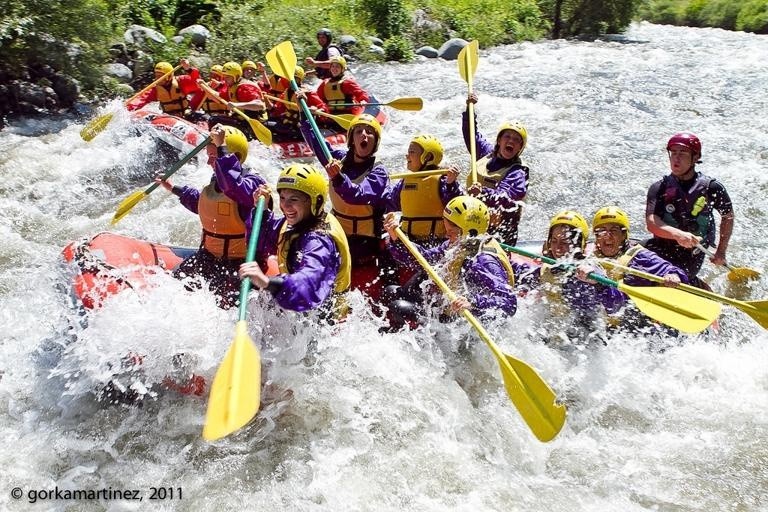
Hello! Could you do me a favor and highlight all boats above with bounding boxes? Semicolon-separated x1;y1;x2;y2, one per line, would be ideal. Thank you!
59;224;547;405
126;110;348;165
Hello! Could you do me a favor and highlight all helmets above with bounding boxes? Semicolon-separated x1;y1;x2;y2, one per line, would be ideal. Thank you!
347;111;382;151
443;194;492;238
666;131;701;158
496;121;528;158
316;28;334;47
276;163;328;218
593;206;630;235
205;124;249;166
548;210;590;252
410;134;444;167
154;55;347;88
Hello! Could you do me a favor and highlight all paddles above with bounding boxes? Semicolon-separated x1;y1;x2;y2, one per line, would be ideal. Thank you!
111;136;213;225
265;41;334;164
328;98;423;111
79;65;181;140
599;260;768;330
382;214;567;443
264;94;355;130
202;194;262;441
696;243;760;281
196;80;273;146
457;40;479;184
499;242;721;333
